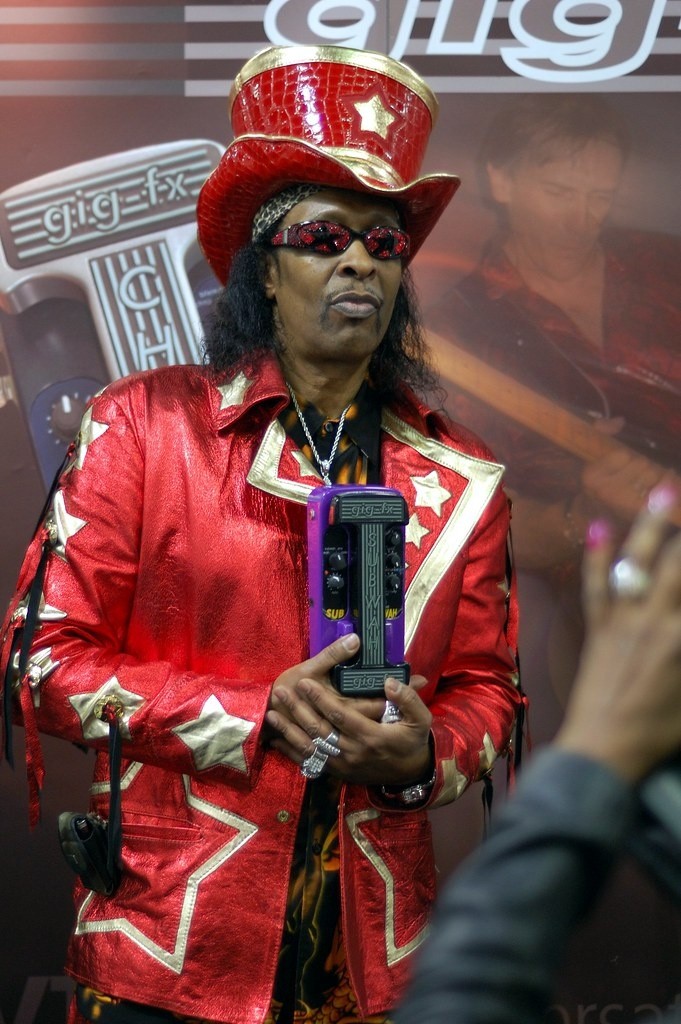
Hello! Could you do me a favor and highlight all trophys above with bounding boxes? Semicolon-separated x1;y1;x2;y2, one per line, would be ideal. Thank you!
307;484;410;697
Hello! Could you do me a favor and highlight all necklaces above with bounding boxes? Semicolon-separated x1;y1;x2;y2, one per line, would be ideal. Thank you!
286;383;352;488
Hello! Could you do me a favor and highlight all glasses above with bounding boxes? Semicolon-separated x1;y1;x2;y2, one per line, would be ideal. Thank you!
262;219;411;260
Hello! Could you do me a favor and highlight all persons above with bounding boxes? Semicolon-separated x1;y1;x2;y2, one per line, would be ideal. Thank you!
0;44;525;1024
429;93;681;473
394;482;680;1024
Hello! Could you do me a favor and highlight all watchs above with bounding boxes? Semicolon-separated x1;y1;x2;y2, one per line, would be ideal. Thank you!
380;770;436;805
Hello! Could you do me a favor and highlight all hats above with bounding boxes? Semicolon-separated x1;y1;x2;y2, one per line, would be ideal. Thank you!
195;44;461;289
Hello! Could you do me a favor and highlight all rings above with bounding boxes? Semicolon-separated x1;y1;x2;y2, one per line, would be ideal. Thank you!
381;701;400;727
611;559;652;597
313;729;341;757
299;749;328;779
